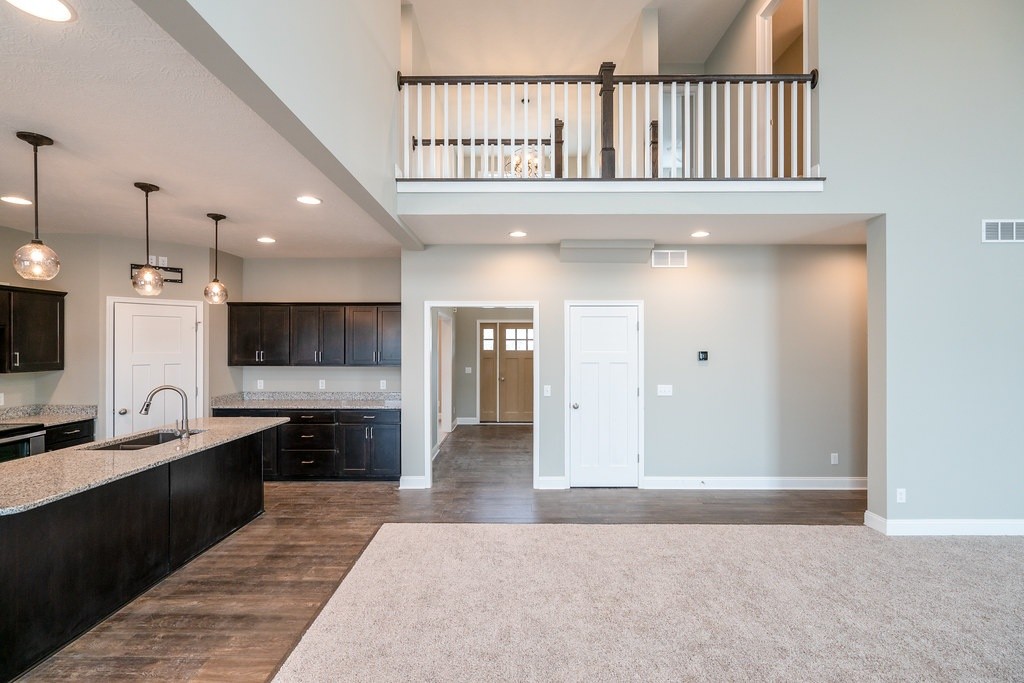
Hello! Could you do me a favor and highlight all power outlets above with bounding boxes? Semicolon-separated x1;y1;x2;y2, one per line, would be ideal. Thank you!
319;380;325;389
897;488;906;502
0;393;5;405
159;256;167;267
149;255;156;266
380;380;386;389
257;380;263;389
831;453;838;464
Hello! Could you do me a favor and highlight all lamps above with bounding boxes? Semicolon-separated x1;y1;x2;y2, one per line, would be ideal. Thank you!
132;182;164;297
344;302;402;367
226;302;289;367
289;301;345;367
13;130;61;281
203;213;228;305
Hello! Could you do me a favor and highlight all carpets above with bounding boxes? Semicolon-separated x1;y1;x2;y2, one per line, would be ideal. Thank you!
264;519;1022;682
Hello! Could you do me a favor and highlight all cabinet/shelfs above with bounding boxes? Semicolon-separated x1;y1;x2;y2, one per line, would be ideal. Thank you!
0;285;69;375
213;409;279;481
336;408;401;482
45;418;95;455
278;409;338;482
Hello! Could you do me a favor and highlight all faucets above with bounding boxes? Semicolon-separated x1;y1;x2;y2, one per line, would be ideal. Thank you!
139;385;189;438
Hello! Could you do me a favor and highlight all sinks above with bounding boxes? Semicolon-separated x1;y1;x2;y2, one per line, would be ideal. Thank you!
125;428;210;446
76;445;148;450
